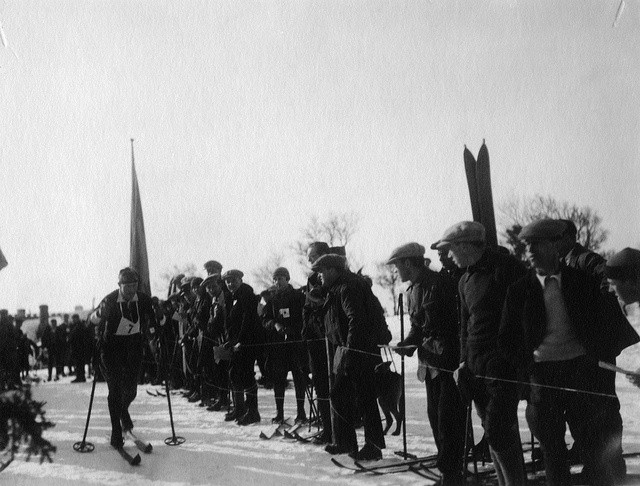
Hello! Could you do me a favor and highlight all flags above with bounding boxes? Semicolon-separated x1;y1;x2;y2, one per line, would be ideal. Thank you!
129;137;151;296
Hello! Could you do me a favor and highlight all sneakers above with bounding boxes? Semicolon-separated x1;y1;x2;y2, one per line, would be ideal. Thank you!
239;408;261;426
214;398;232;411
349;445;382;461
272;417;284;423
324;443;358;456
120;412;133;429
111;429;123;447
313;434;331;445
225;407;247;421
295;414;306;424
188;391;201;402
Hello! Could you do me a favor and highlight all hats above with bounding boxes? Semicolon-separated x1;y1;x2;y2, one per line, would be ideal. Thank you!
190;277;204;288
204;260;223;272
118;266;140;284
311;252;346;271
603;246;639;279
222;270;244;281
178;275;197;288
272;267;290;280
172;274;185;284
518;218;568;242
200;274;220;288
436;221;487;249
387;242;426;266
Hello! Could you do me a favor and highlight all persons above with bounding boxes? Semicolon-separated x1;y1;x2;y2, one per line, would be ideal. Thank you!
199;273;232;411
301;241;332;446
181;277;197;395
36;313;96;383
222;270;261;425
311;254;382;460
98;267;160;444
189;277;211;406
150;293;176;330
385;242;466;486
1;309;36;390
257;268;306;424
555;218;626;477
430;236;462;278
173;274;185;294
204;260;222;276
437;221;527;486
168;293;179;306
498;219;610;486
604;247;640;390
261;290;272;309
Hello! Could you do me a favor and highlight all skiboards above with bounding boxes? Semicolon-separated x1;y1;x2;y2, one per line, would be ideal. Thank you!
404;451;638;484
461;139;505;255
327;441;540;475
113;422;154;467
284;430;325;442
258;416;302;440
